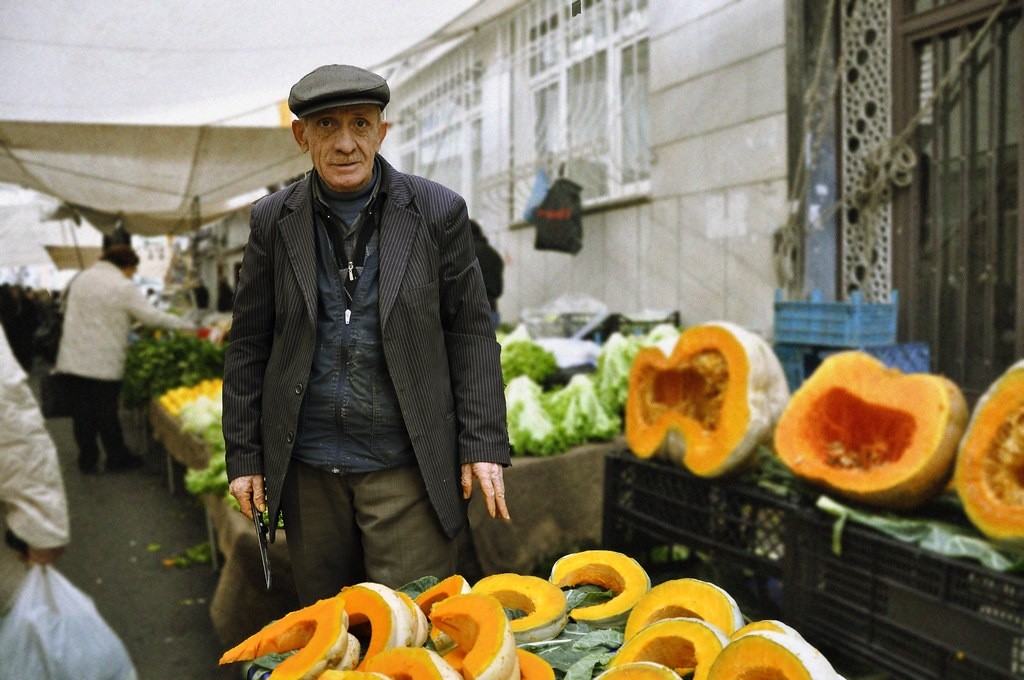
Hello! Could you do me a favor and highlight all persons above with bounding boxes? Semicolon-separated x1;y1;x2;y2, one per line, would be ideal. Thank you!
0;322;68;623
51;228;200;476
223;65;514;608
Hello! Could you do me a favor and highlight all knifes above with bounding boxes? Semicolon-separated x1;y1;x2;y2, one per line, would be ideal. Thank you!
248;494;272;590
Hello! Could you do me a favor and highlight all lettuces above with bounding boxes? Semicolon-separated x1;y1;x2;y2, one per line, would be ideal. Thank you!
498;322;688;456
180;394;285;563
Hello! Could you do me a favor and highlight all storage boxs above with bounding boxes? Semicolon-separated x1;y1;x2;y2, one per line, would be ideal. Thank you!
150;383;207;473
603;287;1024;680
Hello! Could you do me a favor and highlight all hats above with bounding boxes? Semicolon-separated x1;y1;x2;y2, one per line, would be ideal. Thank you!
288;65;390;118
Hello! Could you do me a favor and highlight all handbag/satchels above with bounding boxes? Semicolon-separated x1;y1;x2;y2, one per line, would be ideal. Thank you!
523;170;583;256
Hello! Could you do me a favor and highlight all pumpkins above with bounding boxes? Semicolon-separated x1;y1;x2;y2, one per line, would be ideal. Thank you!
952;358;1024;556
625;320;789;479
216;549;856;680
773;349;968;503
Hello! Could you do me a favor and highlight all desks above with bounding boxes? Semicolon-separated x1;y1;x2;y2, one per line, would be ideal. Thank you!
185;435;626;642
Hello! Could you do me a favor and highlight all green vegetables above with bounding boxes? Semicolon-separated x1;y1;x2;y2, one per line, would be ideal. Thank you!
120;333;230;413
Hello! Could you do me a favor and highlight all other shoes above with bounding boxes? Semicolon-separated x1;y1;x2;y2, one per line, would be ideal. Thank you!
79;458;99;474
105;454;144;472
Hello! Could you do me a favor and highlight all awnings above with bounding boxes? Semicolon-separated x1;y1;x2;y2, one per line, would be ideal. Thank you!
1;119;315;272
